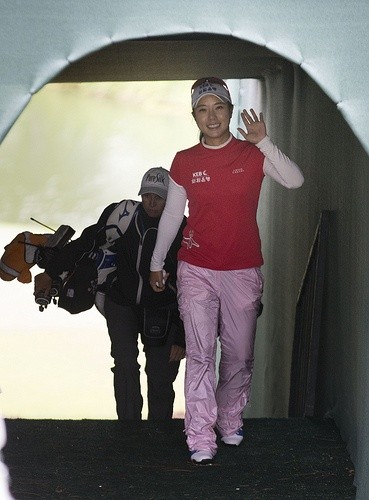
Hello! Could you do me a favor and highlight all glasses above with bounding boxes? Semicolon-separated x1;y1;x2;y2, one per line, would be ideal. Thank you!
191;77;229;94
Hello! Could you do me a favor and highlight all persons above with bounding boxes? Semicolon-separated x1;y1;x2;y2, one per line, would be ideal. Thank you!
150;77;304;465
35;167;188;419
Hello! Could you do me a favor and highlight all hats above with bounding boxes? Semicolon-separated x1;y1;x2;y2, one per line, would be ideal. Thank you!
138;167;170;200
192;82;231;110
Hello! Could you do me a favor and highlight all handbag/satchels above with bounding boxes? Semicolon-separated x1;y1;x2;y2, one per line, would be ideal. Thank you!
58;201;142;317
140;303;172;348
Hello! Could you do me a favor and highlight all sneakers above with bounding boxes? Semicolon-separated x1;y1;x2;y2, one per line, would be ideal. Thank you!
221;427;246;447
191;448;216;466
